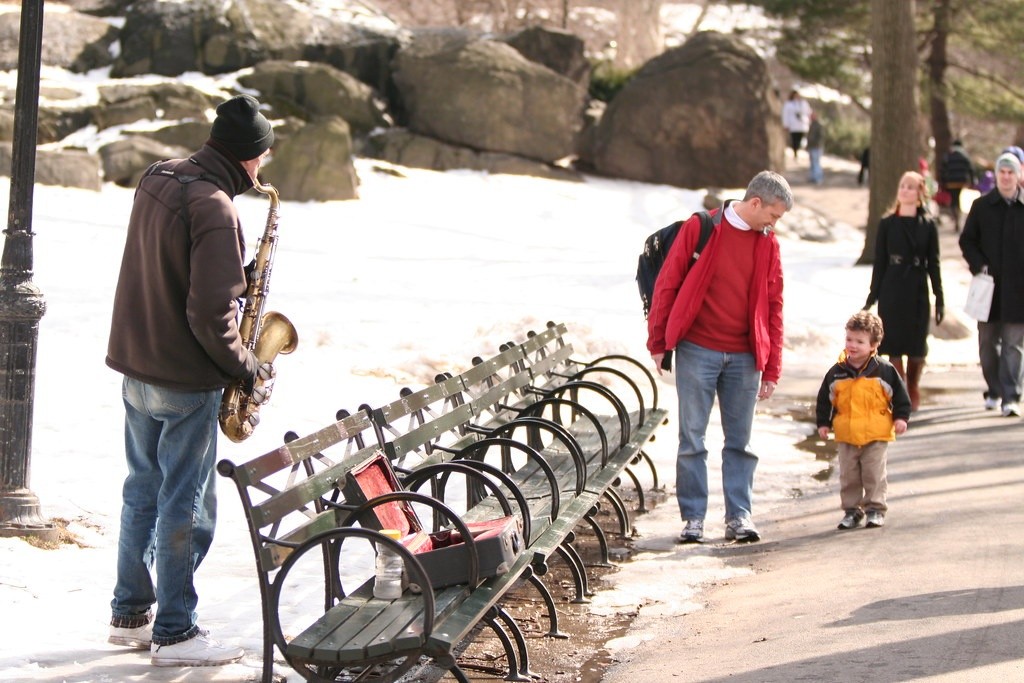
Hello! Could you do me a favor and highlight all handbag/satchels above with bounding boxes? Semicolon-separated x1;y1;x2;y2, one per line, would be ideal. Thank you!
635;210;713;320
964;266;995;323
933;185;953;206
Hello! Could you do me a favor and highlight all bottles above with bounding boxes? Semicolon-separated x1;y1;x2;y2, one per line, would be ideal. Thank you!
373;529;404;599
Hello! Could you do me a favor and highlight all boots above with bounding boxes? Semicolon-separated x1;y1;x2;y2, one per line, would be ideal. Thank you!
889;360;904;379
906;360;924;411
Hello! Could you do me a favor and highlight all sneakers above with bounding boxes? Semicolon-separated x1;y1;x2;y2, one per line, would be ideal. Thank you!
1002;402;1021;418
679;520;705;543
150;629;245;666
107;619;156;650
725;516;760;541
865;511;884;527
837;507;864;528
984;397;1000;410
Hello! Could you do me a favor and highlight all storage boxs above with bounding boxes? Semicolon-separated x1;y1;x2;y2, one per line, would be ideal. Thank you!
334;448;527;592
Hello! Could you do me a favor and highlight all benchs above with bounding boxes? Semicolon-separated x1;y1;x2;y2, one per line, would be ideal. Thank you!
218;323;670;683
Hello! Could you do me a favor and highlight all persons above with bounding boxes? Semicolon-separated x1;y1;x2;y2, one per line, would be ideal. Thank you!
856;135;1024;232
647;171;793;545
815;312;911;530
105;94;276;667
782;90;824;186
958;153;1024;418
863;171;946;412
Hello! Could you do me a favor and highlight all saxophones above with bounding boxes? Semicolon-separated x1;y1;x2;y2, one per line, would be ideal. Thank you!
217;176;300;443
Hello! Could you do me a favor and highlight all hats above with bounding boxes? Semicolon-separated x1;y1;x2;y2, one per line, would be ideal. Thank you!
996;153;1020;179
210;94;274;161
